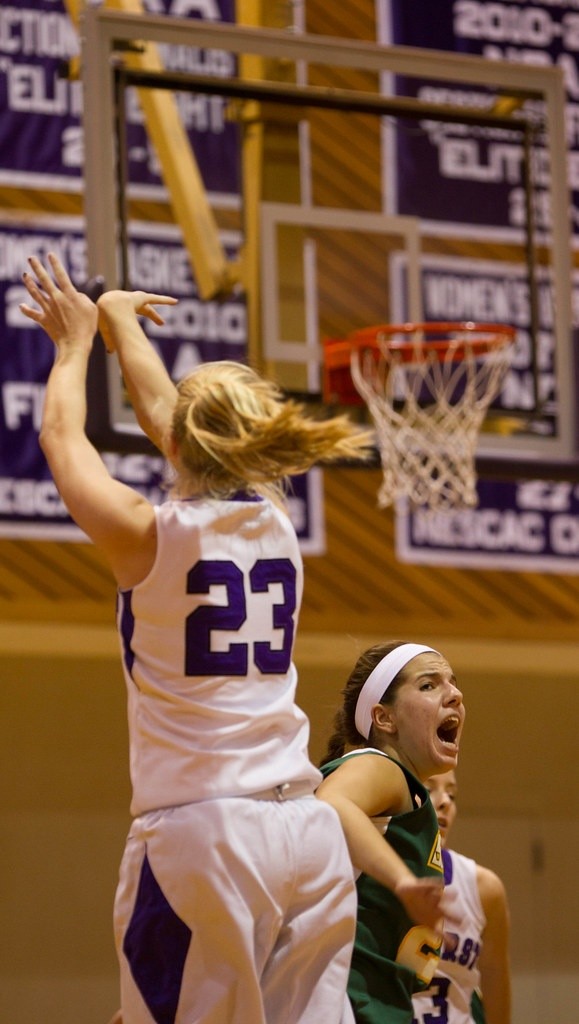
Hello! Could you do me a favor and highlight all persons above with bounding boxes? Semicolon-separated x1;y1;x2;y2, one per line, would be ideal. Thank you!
311;636;515;1024
13;248;360;1024
